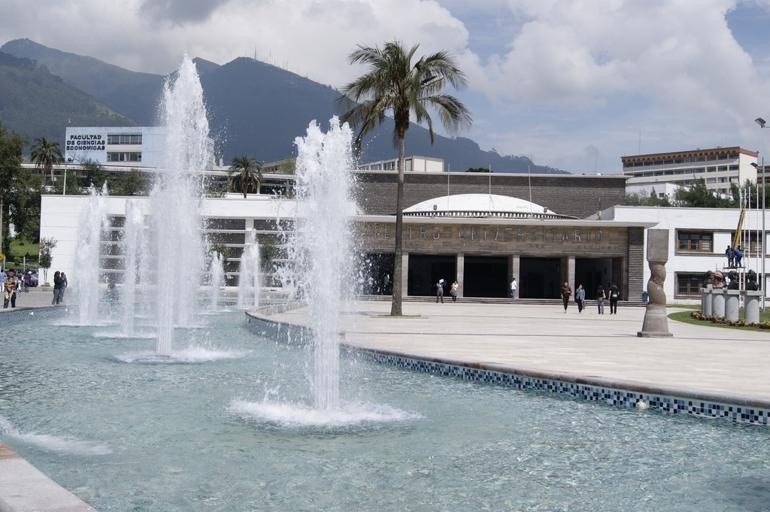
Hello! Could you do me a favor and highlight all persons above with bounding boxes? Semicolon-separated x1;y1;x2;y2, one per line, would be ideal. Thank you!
595;284;606;314
510;277;518;298
725;245;734;267
704;269;758;290
0;269;38;292
608;282;620;314
58;272;69;302
4;270;17;308
575;282;586;312
52;271;63;304
436;278;447;303
559;280;572;311
731;245;743;267
449;280;458;302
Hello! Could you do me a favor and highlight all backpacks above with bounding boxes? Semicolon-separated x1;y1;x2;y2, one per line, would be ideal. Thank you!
612;289;618;299
